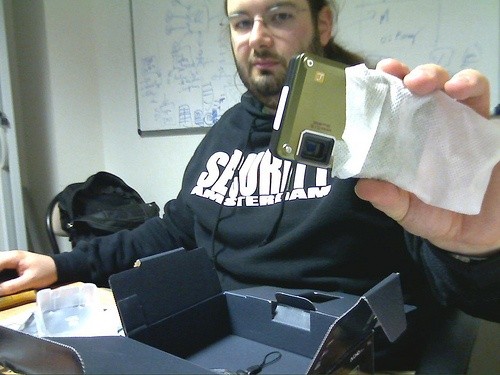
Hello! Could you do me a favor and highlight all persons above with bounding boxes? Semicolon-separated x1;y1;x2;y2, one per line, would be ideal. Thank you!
1;0;500;308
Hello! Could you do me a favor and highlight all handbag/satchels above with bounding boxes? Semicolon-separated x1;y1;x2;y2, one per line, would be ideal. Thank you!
57;170;160;248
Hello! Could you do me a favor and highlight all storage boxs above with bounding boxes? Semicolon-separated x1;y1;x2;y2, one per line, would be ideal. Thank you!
0;245;461;375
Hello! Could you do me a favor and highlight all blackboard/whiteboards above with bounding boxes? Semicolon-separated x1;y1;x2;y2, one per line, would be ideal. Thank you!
128;0;499;137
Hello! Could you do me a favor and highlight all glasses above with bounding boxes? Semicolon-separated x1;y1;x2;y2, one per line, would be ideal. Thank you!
219;6;309;36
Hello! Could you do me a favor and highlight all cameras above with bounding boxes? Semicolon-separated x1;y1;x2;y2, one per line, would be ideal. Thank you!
269;52;436;187
